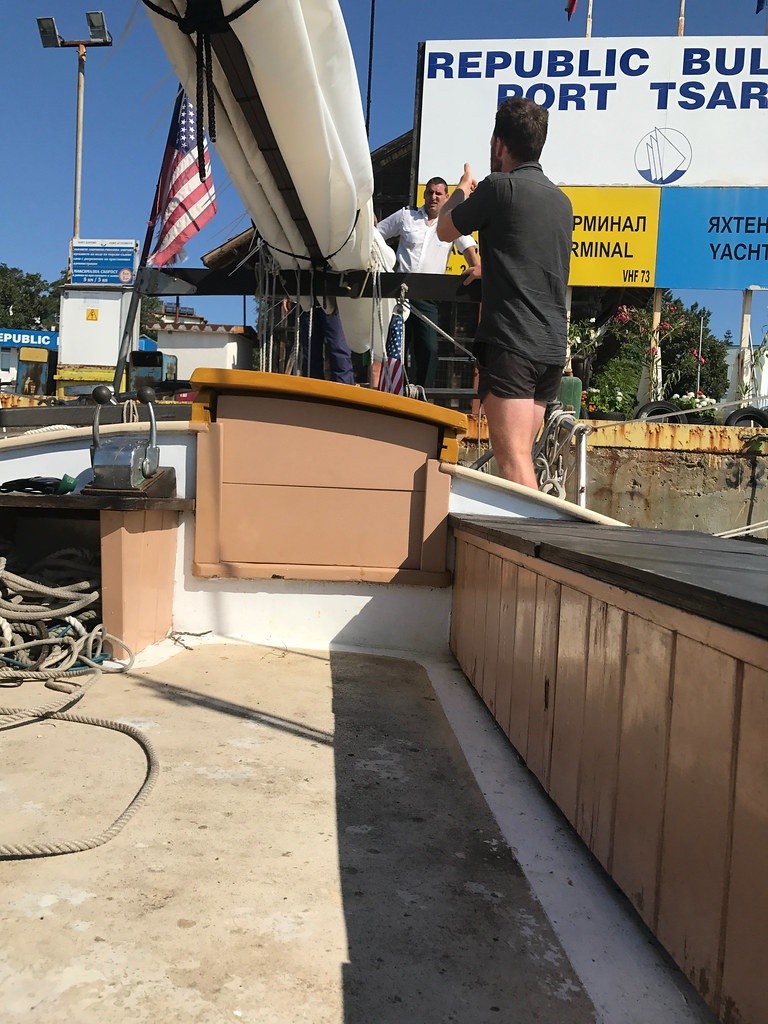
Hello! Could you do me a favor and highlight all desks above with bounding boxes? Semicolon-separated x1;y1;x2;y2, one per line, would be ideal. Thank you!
1;492;195;660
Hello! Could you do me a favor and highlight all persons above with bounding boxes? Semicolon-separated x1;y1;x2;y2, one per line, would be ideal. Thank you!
436;98;573;491
281;289;355;385
377;178;479;388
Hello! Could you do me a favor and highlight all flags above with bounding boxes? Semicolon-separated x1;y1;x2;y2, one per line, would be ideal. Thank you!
378;315;403;395
149;87;218;269
